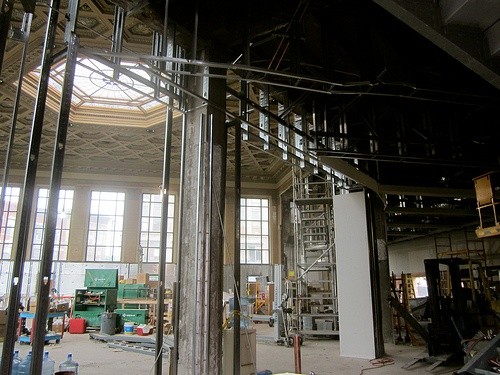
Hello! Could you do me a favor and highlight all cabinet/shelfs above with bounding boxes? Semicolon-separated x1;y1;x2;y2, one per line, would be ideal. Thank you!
73;268;118;327
114;282;148;329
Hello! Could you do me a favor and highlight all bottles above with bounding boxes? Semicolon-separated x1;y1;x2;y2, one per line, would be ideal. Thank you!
17;351;32;375
42;352;55;375
11;351;22;375
59;354;78;375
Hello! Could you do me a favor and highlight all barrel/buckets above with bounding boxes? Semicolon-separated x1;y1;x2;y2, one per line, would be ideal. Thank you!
70;318;86;334
229;297;249;328
124;322;134;335
101;313;116;335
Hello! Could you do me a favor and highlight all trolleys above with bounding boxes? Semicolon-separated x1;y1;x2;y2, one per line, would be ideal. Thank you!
276;305;303;346
